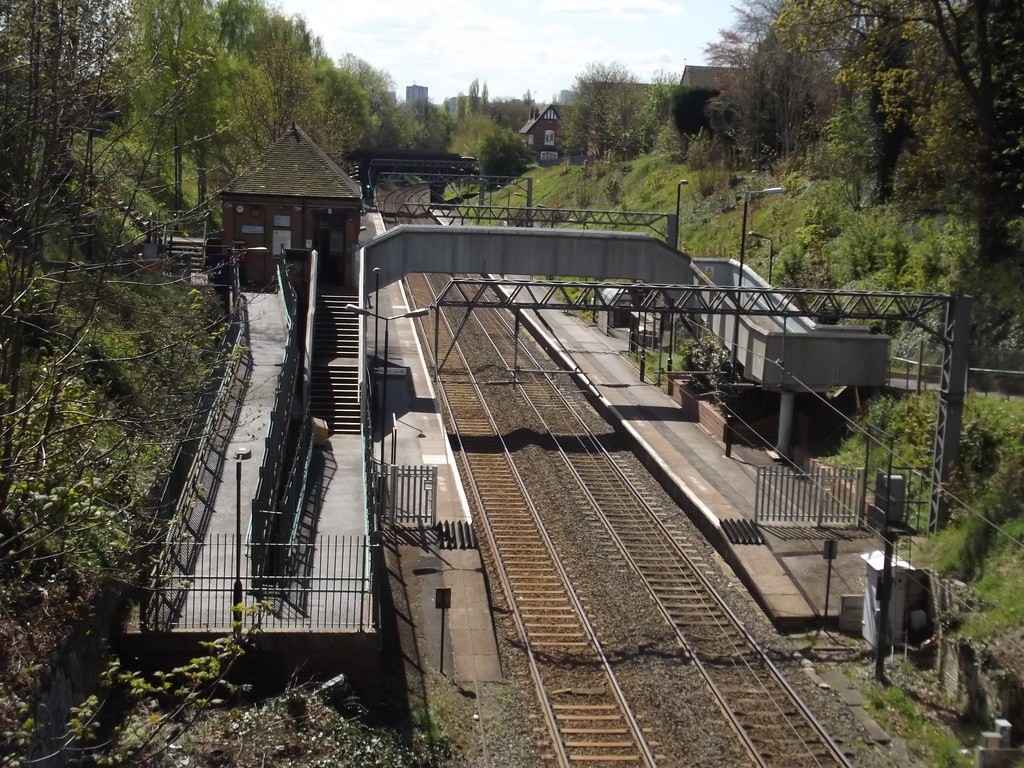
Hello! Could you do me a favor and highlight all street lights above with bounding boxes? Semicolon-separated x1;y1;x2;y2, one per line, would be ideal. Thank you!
495;184;510;225
234;447;252;640
746;230;773;287
732;187;785;383
675;180;689;250
